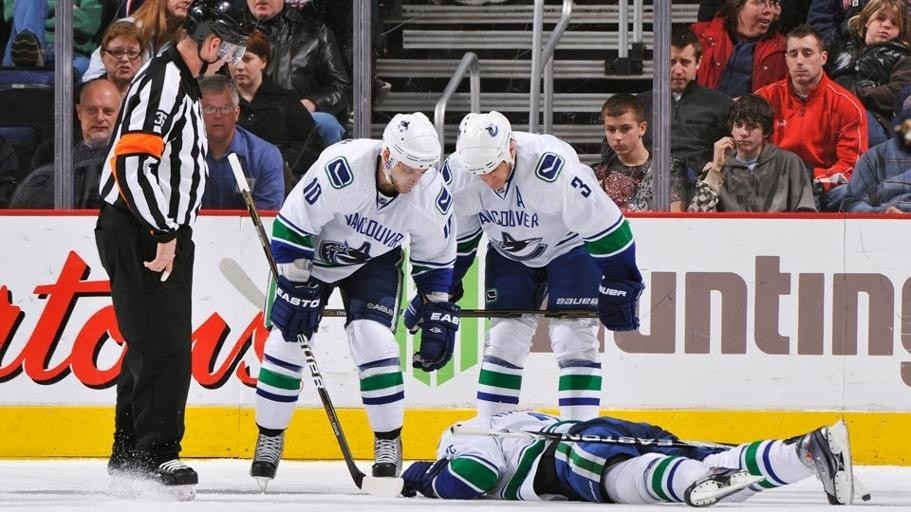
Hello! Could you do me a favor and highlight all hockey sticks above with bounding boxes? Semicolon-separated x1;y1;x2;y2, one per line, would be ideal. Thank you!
449;425;873;503
219;256;604;319
228;154;405;499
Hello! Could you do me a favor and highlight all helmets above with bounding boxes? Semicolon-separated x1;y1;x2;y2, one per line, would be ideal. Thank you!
181;0;256;65
456;111;515;175
380;112;442;169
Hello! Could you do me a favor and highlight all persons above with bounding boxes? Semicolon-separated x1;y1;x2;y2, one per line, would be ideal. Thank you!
401;410;834;507
94;0;249;485
2;2;353;210
251;112;457;475
589;1;911;212
404;111;644;422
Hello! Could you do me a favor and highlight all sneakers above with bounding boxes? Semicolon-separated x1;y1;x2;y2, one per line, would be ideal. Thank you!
684;467;738;507
252;432;283;477
795;426;840;504
372;435;402;477
109;446;197;485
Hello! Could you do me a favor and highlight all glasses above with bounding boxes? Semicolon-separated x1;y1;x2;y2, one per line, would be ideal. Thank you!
203;105;234;114
104;49;141;60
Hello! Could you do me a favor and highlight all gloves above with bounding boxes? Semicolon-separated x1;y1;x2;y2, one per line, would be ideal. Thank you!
271;275;320;342
598;274;645;330
401;458;448;497
404;289;461;371
